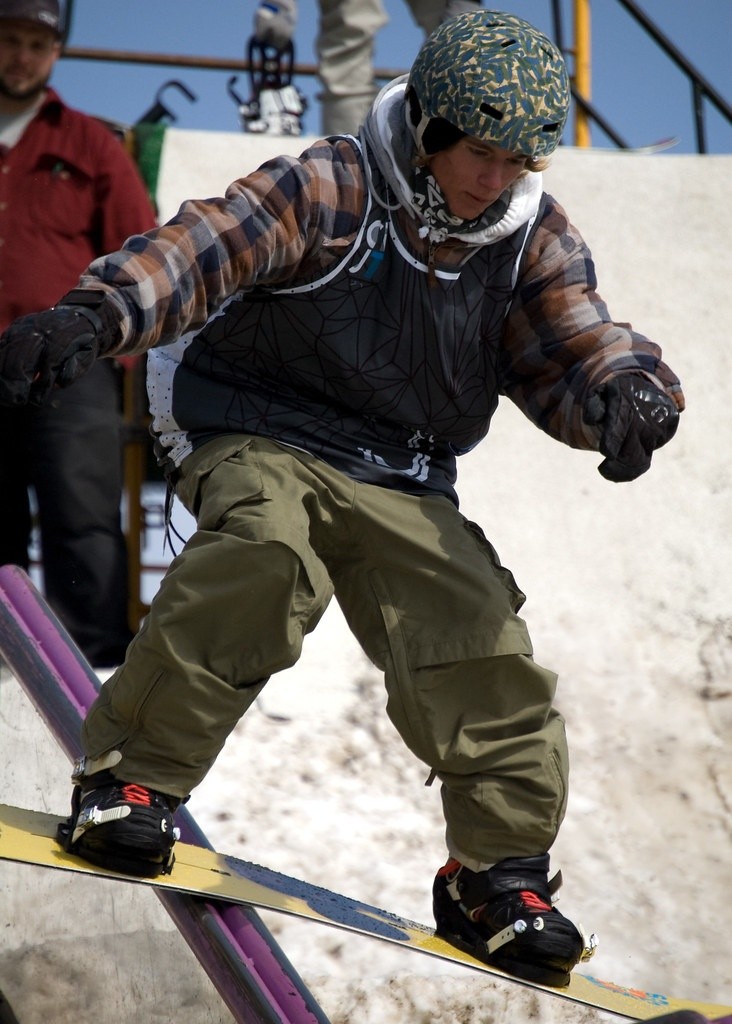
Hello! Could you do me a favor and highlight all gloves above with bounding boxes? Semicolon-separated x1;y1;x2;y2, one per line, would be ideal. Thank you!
1;288;123;415
582;370;680;483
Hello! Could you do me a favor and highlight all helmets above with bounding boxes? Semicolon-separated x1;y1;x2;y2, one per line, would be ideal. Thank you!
404;8;571;172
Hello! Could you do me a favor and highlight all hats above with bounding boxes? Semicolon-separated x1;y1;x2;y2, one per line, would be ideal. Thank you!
0;0;72;39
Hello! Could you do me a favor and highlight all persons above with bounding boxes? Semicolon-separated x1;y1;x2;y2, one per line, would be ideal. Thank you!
0;0;159;667
0;15;686;986
250;0;498;146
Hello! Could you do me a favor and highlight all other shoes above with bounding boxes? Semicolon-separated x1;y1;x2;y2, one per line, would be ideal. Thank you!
66;777;177;865
432;853;585;974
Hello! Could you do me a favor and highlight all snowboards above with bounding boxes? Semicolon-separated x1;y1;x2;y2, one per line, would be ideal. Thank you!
0;803;731;1023
